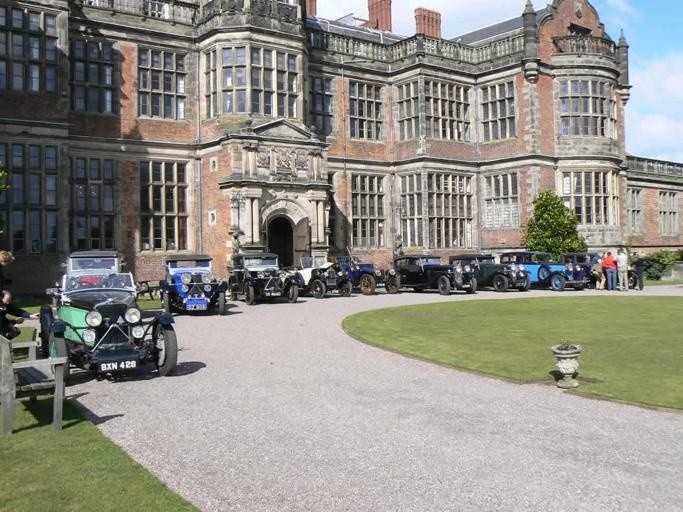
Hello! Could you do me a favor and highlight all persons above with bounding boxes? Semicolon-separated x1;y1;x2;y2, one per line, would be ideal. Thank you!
0;250;15;312
629;251;644;291
590;250;606;290
613;248;629;291
0;289;39;340
602;251;617;291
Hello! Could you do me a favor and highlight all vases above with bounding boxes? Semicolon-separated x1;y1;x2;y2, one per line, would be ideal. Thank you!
552;345;582;388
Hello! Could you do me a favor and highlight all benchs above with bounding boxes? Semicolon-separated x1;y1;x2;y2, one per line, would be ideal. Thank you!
0;335;68;435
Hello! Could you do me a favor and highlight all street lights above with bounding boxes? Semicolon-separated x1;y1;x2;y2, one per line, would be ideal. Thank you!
230;188;245;244
